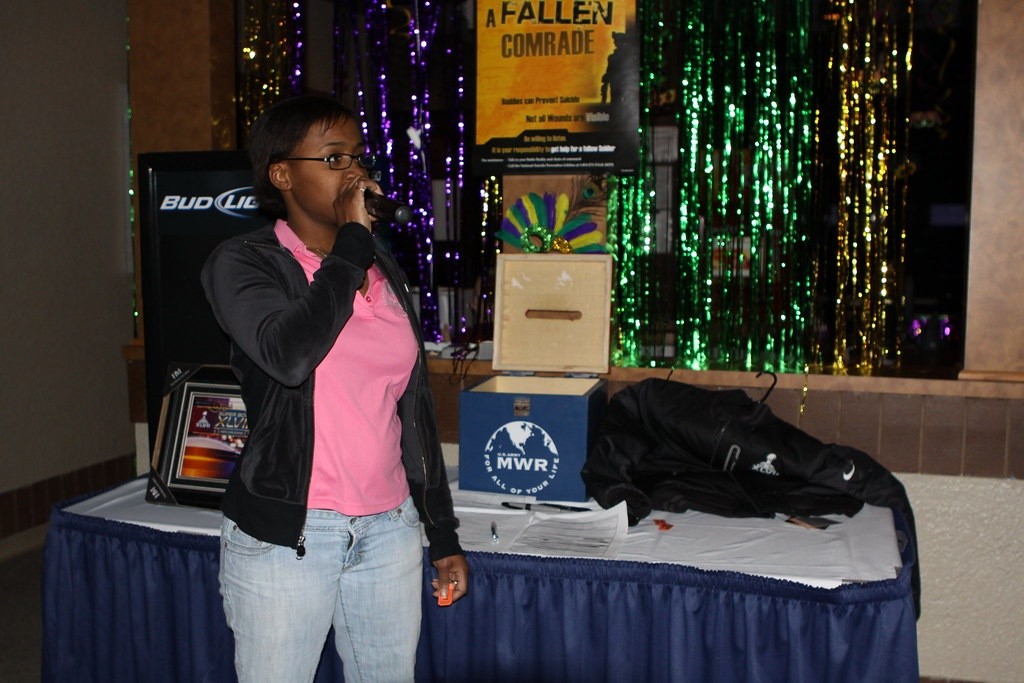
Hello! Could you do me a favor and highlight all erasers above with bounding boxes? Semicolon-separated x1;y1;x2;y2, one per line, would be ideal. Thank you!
438;583;453;606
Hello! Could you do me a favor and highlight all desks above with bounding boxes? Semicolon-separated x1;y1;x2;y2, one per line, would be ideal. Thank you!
38;442;922;683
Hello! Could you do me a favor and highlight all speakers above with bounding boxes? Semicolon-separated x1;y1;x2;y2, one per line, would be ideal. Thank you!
136;150;288;470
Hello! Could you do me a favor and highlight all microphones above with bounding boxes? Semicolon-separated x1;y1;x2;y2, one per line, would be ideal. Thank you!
364;189;414;224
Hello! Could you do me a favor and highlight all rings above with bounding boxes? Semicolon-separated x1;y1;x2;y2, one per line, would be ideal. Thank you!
454;582;457;584
360;189;366;192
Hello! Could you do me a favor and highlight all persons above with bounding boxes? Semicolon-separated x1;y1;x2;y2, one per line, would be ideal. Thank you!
198;89;470;683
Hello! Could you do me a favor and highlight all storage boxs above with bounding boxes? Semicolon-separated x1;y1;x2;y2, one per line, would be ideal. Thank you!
456;254;614;502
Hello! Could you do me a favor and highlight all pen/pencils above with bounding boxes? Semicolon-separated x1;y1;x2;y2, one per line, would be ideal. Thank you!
502;502;561;513
491;522;499;542
540;503;592;512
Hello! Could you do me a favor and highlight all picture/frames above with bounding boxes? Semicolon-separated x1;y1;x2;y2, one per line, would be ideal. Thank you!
144;363;265;509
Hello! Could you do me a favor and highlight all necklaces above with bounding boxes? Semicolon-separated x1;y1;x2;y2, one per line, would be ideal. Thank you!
308;246;328;257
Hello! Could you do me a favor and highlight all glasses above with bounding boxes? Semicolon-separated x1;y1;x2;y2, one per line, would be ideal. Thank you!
281;153;376;170
